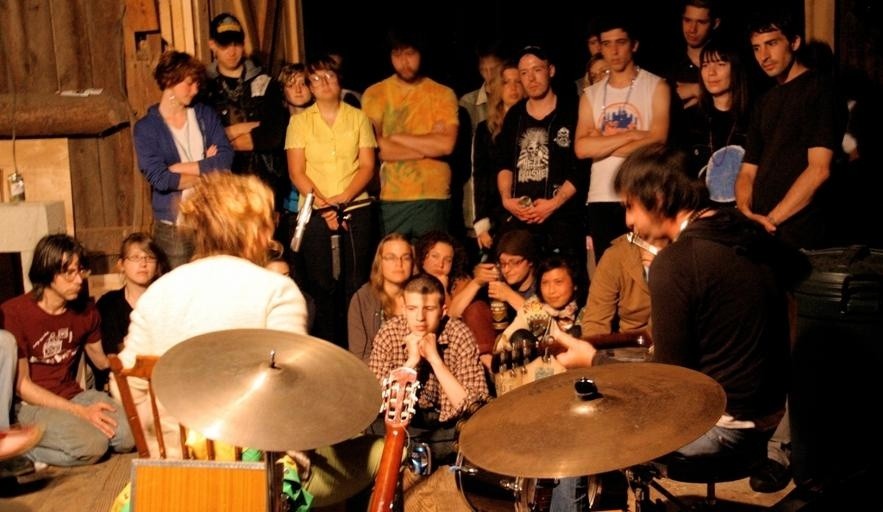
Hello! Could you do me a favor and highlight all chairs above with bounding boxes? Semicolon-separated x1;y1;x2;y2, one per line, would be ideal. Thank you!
105;352;284;512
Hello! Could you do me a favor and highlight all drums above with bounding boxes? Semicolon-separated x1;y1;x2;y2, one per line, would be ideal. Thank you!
455;451;535;512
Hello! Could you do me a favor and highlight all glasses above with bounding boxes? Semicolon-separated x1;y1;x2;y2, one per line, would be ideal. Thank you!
312;71;336;85
496;256;526;269
381;254;412;264
62;267;92;282
124;253;157;264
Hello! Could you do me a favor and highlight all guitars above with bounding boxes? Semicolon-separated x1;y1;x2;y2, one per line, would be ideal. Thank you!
367;367;422;511
492;328;653;372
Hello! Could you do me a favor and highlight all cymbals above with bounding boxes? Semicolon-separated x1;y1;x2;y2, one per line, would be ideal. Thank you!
151;329;382;453
458;360;729;479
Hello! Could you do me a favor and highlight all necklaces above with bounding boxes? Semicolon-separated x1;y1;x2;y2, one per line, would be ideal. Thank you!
602;66;641;133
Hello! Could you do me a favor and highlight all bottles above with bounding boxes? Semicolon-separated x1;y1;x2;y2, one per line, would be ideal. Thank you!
490;263;508;333
411;443;430;477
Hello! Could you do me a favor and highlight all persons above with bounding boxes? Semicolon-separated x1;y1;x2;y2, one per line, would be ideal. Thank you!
534;254;578;327
265;254;292;277
457;51;502;270
417;234;454;313
576;23;670;251
450;232;537;323
0;235;135;468
668;3;722;110
137;50;233;267
109;176;306;462
475;63;525;257
679;42;746;205
0;328;46;477
252;63;312;296
550;141;791;469
203;13;285;208
578;27;601;101
285;55;378;334
370;273;489;469
497;43;576;254
348;234;413;359
581;228;670;361
736;14;836;233
362;36;458;236
95;232;163;391
588;53;610;84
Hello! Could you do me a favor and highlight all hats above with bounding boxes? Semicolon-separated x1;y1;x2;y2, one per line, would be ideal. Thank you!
515;44;548;60
209;11;246;43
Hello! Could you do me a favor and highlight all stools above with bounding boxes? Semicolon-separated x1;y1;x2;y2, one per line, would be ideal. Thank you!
666;444;768;511
